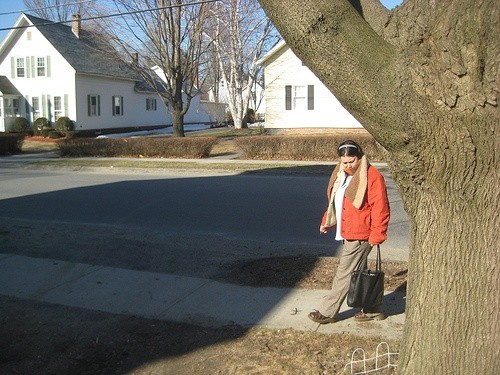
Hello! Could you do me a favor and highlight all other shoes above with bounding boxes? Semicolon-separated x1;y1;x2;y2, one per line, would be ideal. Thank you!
307;311;336;323
354;311;386;322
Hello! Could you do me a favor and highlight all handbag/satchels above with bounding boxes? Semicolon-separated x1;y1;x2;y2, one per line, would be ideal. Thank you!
347;242;385;307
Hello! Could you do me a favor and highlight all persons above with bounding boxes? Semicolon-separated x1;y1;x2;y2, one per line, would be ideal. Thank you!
307;140;391;325
